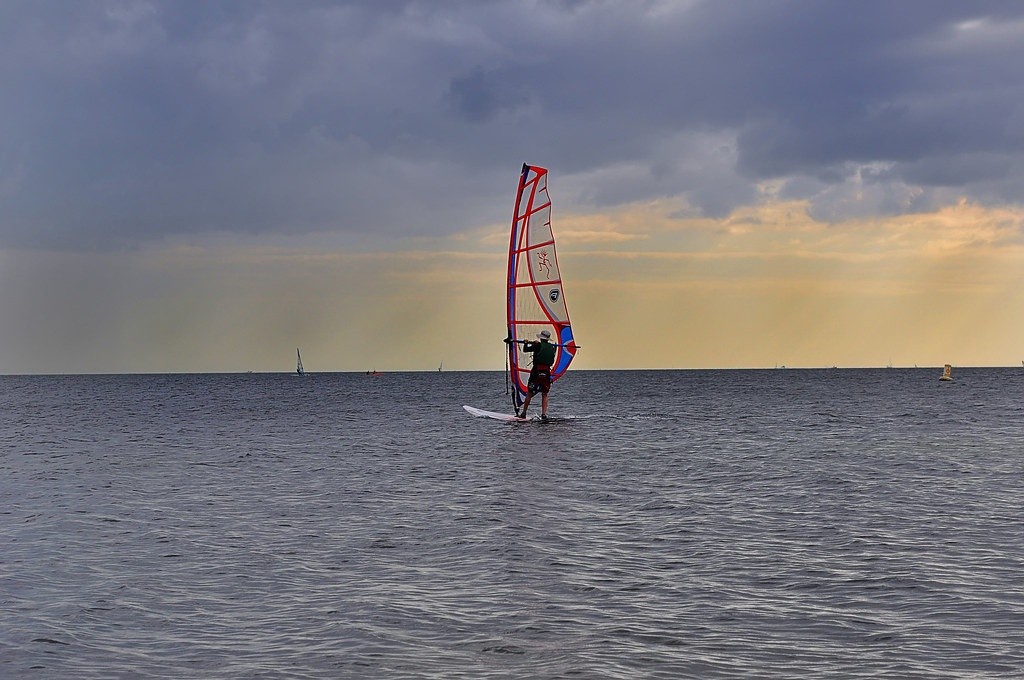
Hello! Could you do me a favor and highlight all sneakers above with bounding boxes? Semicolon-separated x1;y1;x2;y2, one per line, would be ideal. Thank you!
519;413;526;418
541;414;548;420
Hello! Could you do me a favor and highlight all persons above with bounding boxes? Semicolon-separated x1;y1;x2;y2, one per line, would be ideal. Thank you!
518;330;557;418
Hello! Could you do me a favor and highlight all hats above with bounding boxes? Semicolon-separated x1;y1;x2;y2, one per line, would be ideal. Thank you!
536;331;554;341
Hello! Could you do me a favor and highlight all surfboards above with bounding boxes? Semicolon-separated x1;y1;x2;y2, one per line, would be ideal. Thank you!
462;405;532;421
